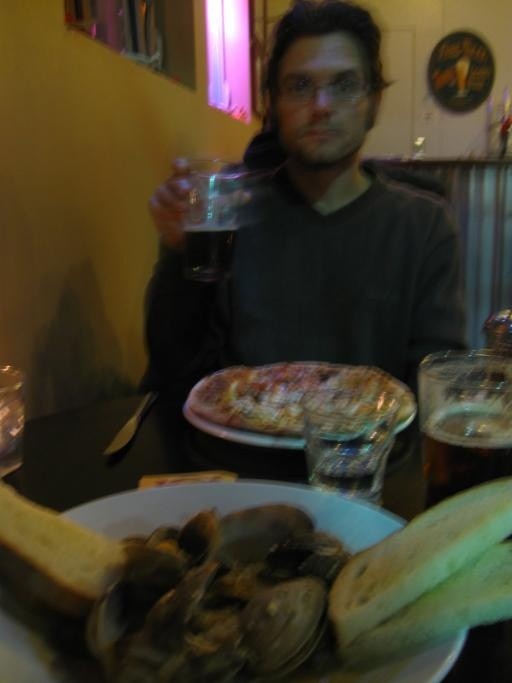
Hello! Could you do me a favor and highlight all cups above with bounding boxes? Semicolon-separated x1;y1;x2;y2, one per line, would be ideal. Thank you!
179;156;246;282
0;365;26;477
484;90;510;158
300;386;400;506
418;348;512;514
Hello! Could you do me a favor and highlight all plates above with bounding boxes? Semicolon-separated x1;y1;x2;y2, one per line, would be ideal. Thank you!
1;475;477;683
182;358;418;452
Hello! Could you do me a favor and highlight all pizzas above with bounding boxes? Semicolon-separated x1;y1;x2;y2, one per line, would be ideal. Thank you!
188;361;416;439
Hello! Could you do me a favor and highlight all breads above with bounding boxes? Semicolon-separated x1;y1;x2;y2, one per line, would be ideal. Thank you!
326;474;512;670
0;482;126;619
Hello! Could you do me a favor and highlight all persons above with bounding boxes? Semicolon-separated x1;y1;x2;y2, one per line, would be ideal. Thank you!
143;2;464;399
